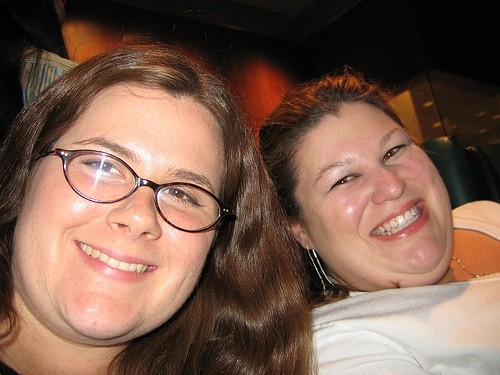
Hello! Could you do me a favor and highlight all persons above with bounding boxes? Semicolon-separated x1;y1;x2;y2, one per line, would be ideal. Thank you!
257;65;500;375
0;37;315;375
0;0;81;147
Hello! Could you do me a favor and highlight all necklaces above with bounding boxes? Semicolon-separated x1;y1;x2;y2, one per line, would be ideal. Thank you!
451;256;492;278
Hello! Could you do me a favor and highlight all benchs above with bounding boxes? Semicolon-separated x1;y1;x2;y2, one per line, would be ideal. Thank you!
418;144;500;207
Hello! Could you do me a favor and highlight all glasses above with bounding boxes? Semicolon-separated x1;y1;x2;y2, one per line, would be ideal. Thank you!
29;148;236;233
